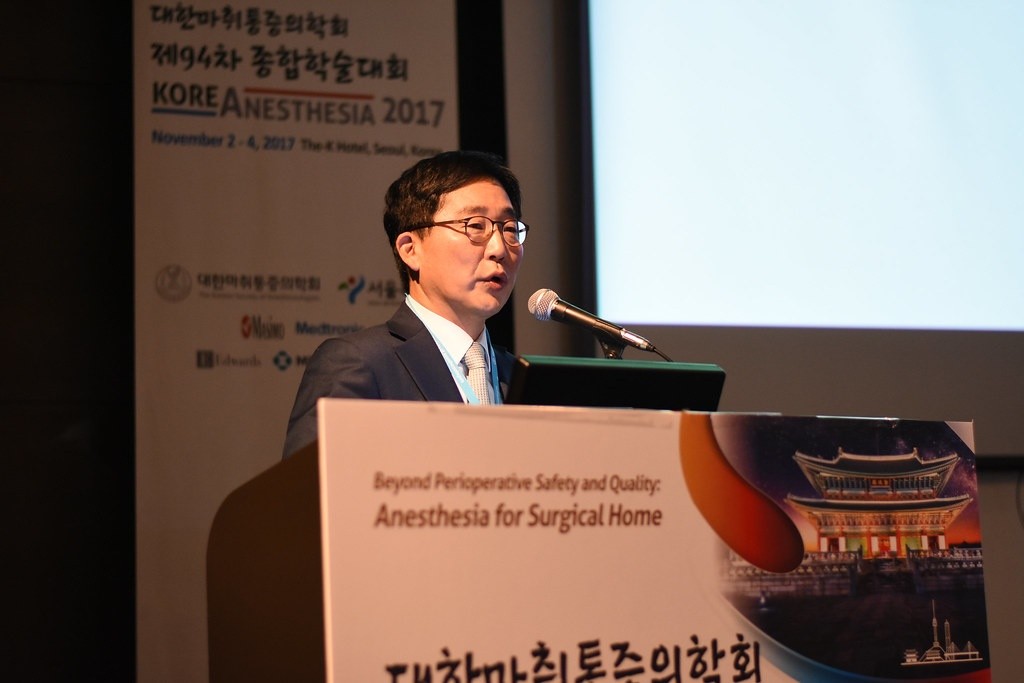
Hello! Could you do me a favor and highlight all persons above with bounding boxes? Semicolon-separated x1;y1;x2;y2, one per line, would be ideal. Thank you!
281;151;531;464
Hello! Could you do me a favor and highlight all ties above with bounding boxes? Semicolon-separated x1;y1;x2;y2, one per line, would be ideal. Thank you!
461;342;491;405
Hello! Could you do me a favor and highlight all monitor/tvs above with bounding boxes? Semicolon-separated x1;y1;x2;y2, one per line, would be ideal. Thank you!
504;354;726;412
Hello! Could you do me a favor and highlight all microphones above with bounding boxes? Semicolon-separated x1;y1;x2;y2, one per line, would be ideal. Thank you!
528;288;656;353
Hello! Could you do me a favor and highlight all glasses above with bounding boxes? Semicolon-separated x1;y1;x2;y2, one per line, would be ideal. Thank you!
404;216;530;247
